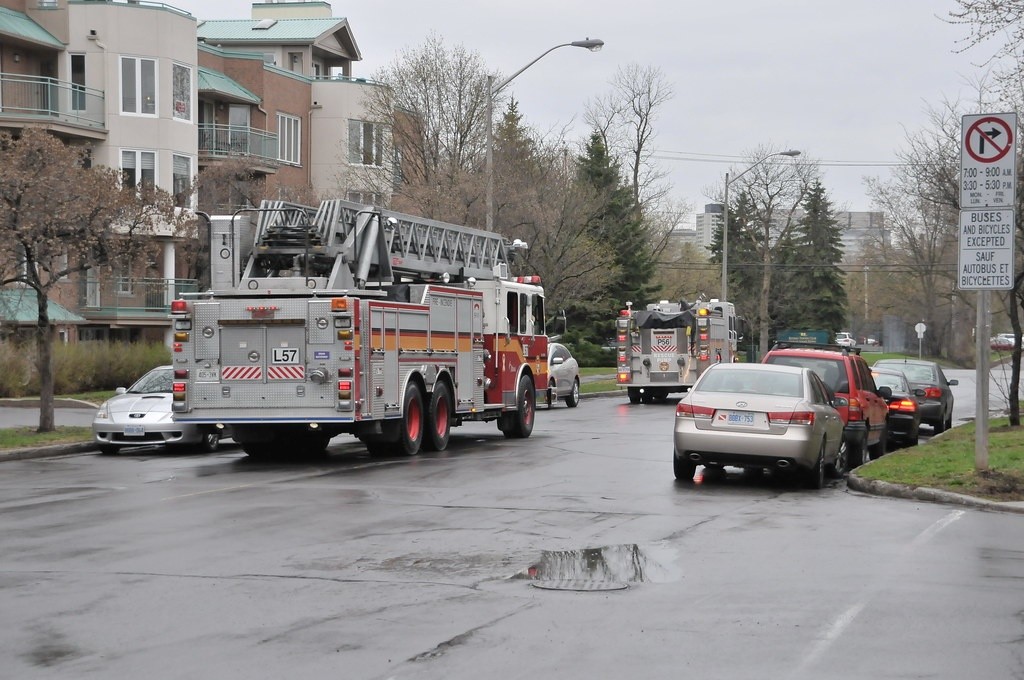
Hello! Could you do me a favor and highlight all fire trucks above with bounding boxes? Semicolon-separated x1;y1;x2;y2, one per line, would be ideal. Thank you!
617;293;745;402
169;198;566;461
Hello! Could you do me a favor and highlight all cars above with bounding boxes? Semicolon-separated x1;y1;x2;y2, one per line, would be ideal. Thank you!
673;363;850;490
546;341;582;408
867;366;927;449
873;358;959;435
989;337;1013;349
834;332;857;347
864;335;879;345
92;364;240;454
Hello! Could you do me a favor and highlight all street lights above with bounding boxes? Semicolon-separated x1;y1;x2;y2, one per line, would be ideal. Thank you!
720;150;801;304
485;37;605;231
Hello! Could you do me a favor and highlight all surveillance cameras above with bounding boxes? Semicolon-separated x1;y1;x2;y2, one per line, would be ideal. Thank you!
90;29;97;36
314;101;318;105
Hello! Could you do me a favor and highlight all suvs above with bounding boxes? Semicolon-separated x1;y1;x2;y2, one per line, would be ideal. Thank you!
760;340;893;469
